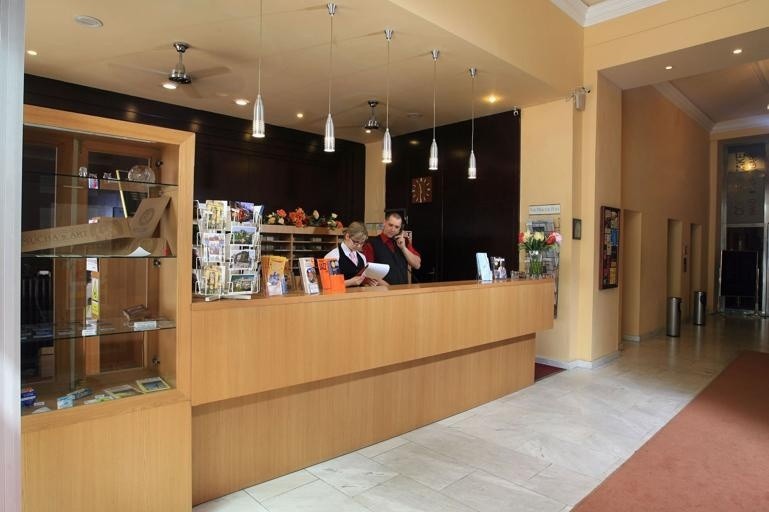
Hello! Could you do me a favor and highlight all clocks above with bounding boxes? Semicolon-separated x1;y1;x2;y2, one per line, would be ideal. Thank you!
407;174;435;208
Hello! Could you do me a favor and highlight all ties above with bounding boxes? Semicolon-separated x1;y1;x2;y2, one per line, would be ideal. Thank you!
350;251;357;266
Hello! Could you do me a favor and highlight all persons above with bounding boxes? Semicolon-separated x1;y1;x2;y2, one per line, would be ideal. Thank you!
324;222;388;288
363;214;421;285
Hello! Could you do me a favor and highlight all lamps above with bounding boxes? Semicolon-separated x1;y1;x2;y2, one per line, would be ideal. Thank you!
323;2;337;153
381;28;394;164
468;67;476;180
428;48;440;170
252;0;265;138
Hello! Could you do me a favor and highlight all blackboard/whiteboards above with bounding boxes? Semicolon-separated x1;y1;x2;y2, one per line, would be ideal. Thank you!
719;249;758;299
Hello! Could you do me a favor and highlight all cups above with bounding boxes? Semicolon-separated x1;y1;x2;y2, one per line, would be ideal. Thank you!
79;167;87;177
511;271;519;281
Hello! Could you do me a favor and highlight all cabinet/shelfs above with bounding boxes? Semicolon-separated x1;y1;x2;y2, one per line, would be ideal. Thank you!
262;224;413;285
21;103;196;511
193;200;263;302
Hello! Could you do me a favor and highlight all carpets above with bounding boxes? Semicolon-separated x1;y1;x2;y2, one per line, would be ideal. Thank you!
568;350;768;512
535;363;566;382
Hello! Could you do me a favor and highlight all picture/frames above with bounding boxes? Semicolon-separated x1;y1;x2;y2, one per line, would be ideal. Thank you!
599;206;620;290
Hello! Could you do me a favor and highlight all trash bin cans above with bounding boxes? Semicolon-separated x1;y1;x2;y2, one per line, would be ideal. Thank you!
694;291;705;325
666;297;681;337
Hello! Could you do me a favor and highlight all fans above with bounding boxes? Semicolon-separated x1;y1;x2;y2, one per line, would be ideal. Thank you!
122;41;232;98
335;100;416;143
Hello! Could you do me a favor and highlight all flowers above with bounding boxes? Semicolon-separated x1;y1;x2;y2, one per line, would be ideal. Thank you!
266;206;344;230
515;230;558;250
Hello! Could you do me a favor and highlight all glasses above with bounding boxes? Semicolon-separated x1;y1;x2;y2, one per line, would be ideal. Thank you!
349;236;365;246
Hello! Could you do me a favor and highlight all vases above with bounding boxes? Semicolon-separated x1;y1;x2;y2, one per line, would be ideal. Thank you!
527;250;542;280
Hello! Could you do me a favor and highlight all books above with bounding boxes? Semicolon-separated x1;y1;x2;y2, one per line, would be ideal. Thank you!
104;375;171;398
199;201;285;296
317;259;347;290
299;258;319;295
357;262;390;285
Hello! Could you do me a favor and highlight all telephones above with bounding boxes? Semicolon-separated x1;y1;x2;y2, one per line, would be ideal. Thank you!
392;222;405;241
